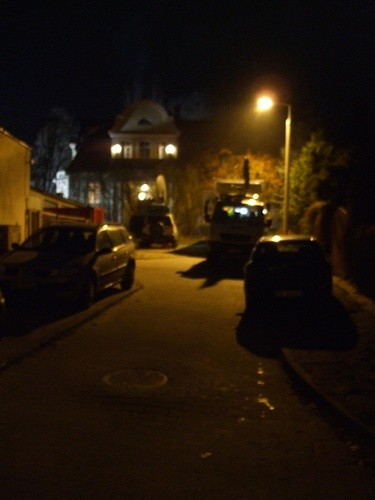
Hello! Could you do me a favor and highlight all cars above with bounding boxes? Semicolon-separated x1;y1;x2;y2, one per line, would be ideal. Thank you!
242;234;333;309
0;224;138;310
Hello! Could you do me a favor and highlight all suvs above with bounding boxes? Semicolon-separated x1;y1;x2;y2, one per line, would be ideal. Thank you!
139;210;180;248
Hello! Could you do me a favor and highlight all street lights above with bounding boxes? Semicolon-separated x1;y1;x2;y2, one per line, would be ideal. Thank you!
256;95;292;235
